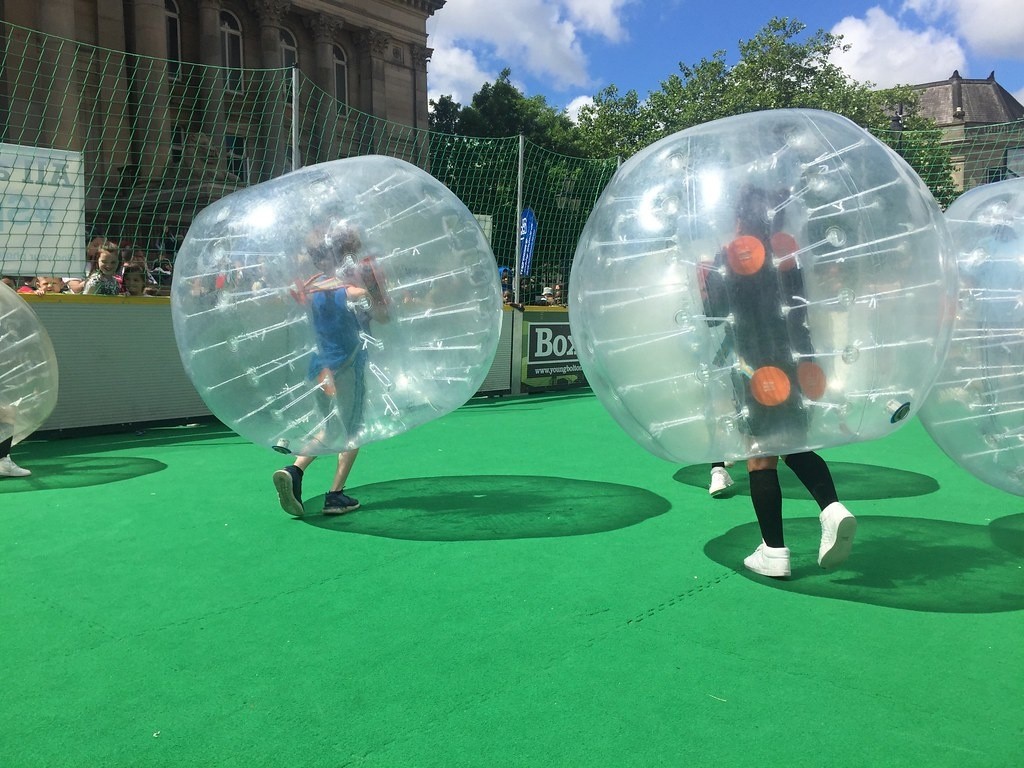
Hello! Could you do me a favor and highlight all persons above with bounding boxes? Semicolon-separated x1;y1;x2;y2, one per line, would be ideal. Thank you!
0;404;32;477
0;225;442;306
697;183;856;577
496;266;565;311
273;223;392;518
698;368;737;497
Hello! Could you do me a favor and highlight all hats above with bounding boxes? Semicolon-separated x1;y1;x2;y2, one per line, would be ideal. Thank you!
541;286;554;296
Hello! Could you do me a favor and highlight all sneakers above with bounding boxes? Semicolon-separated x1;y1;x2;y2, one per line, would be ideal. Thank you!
274;465;306;516
744;538;791;577
322;489;361;514
710;467;734;495
817;502;857;571
1;454;31;476
